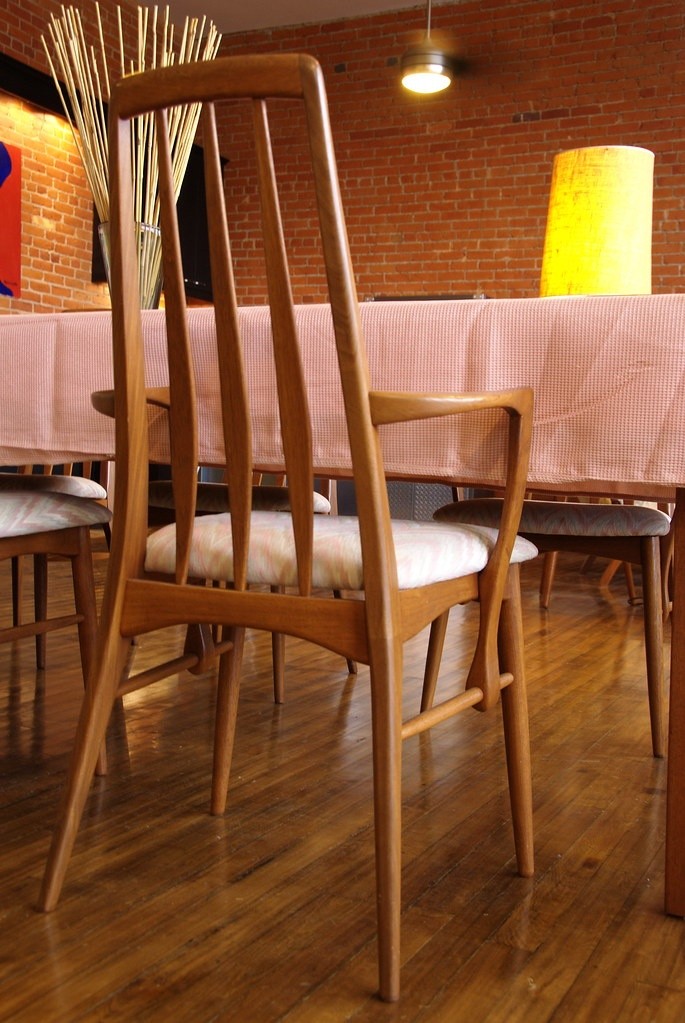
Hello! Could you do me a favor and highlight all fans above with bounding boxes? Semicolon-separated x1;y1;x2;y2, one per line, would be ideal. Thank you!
342;0;524;94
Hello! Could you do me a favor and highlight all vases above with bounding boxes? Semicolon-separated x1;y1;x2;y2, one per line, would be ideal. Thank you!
95;222;165;314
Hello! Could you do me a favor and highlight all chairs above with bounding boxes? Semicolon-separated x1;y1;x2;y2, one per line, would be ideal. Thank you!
36;52;538;998
0;461;115;773
148;461;358;703
416;468;676;757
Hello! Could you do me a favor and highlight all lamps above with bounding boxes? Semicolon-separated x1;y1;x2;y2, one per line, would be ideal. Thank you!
400;65;454;95
540;146;655;297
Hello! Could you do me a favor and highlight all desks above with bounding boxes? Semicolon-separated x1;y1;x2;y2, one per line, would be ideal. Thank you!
0;310;684;918
